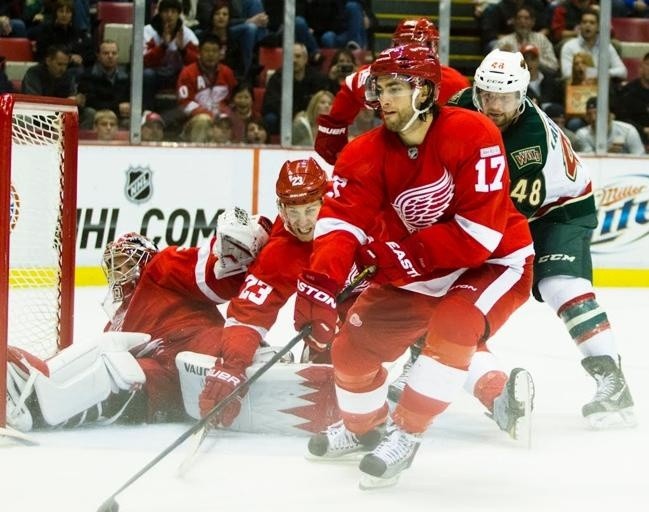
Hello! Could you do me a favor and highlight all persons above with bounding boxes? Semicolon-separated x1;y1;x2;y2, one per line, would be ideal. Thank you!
472;0;649;156
294;44;534;478
198;157;535;440
5;205;340;432
315;18;470;166
1;1;376;142
388;44;633;417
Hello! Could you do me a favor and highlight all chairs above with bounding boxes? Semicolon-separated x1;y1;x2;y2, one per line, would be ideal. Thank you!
612;18;649;43
11;78;23;94
102;22;133;64
96;2;135;34
259;47;283;87
6;20;26;38
319;47;362;77
4;61;38;80
623;59;643;80
254;87;280;143
618;41;649;59
0;38;33;61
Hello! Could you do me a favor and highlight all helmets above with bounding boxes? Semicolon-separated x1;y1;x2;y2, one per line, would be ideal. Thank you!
472;49;531;115
391;17;442;56
101;232;160;304
366;44;442;102
276;157;328;238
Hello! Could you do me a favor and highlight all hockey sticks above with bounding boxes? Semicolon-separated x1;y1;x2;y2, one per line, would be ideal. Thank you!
98;265;378;512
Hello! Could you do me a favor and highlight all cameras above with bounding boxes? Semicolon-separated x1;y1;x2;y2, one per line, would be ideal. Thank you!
338;64;354;74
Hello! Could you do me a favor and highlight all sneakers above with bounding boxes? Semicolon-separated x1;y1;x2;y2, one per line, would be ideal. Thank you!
580;355;632;428
360;424;423;489
484;367;535;448
386;345;421;414
306;420;382;461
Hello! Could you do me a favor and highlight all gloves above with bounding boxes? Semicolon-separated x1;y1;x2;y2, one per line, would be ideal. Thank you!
294;267;338;353
355;236;433;285
315;115;348;165
199;351;252;427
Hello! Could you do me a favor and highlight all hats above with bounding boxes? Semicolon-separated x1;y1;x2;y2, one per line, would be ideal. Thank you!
140;113;166;128
519;44;538;55
209;111;233;126
586;96;597;109
